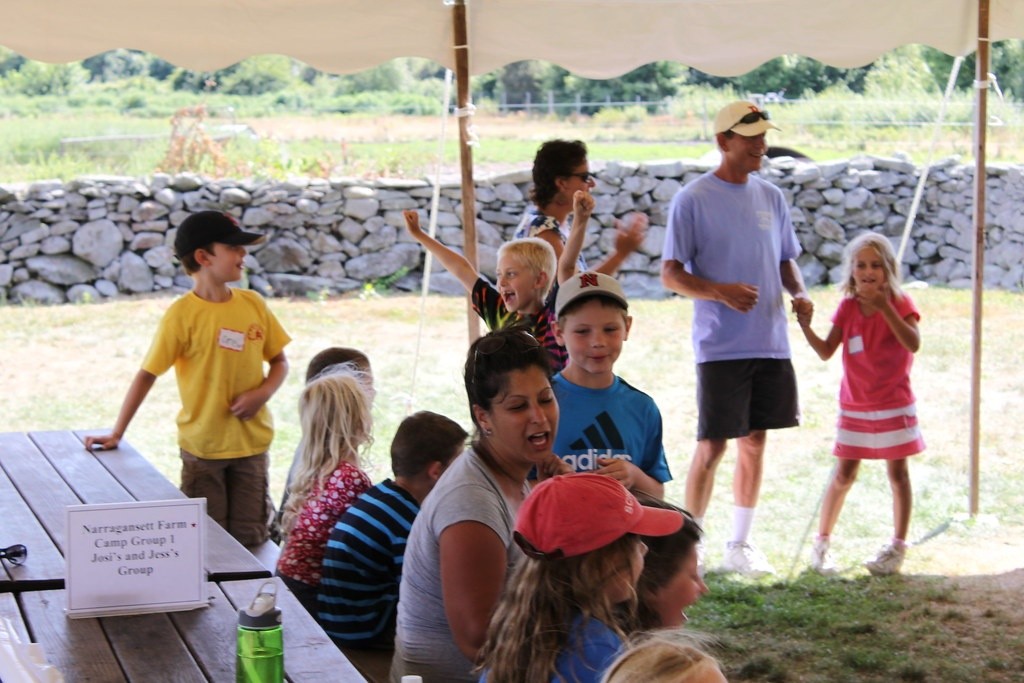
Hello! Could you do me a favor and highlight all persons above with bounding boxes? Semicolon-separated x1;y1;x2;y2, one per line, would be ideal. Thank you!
316;410;469;652
660;101;814;580
85;210;292;547
796;231;926;576
511;139;649;276
469;431;533;494
402;189;595;378
274;347;376;622
469;472;728;683
526;270;673;537
387;328;576;683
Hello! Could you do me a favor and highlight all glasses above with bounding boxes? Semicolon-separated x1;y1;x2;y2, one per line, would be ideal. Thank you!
725;111;771;132
571;171;590;182
470;331;540;399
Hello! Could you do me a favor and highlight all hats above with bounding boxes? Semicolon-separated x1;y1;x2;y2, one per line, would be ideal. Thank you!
512;472;684;555
173;211;265;259
714;102;782;137
555;271;628;321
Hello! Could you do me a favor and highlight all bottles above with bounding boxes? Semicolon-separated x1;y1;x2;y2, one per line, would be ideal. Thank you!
236;580;283;683
401;675;423;683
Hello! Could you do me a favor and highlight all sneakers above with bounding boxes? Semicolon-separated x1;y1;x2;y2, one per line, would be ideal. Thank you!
866;544;906;575
722;539;776;579
694;542;706;580
810;534;837;576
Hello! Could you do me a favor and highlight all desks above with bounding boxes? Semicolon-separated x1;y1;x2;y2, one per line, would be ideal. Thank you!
0;428;366;683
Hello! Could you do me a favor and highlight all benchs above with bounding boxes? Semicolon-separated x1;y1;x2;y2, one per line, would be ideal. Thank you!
241;533;396;683
